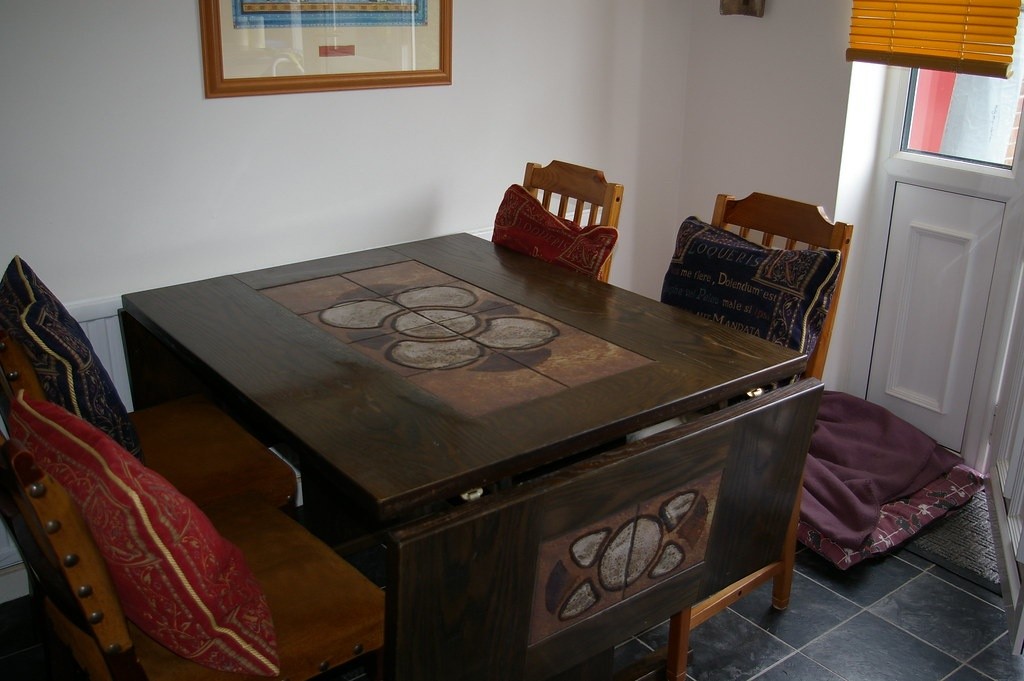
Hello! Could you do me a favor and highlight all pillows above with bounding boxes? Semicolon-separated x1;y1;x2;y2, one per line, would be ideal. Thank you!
9;388;281;679
660;215;842;414
491;185;620;277
0;255;138;457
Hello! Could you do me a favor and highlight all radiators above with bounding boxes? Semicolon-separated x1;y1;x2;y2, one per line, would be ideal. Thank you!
0;296;126;568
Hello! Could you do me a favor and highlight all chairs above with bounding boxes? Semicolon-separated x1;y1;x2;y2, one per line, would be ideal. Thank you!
523;159;624;281
0;320;299;525
625;191;854;446
0;432;385;681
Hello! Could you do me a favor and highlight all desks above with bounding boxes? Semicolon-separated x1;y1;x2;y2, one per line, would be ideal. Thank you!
119;232;825;681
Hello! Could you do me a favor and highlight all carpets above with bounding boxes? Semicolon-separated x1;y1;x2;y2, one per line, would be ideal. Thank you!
907;492;1003;599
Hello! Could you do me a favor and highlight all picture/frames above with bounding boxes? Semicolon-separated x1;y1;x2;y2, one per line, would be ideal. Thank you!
199;0;453;99
